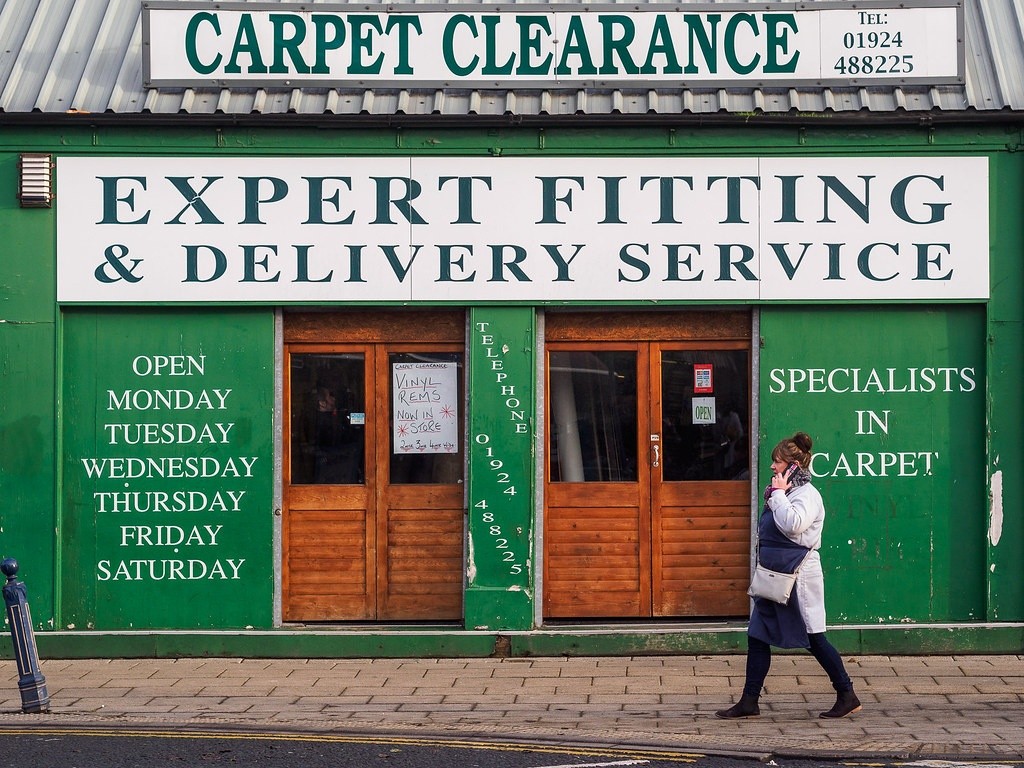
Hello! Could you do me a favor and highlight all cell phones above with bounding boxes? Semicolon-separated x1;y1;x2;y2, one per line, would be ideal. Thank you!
782;462;801;484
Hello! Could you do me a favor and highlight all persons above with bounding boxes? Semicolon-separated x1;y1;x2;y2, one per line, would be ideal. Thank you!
712;399;749;481
715;433;863;719
307;386;338;416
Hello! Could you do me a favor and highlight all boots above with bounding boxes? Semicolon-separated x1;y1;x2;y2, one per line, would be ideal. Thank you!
819;682;862;719
715;689;762;719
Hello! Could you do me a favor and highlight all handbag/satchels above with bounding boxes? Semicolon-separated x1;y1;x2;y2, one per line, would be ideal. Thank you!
746;562;797;606
